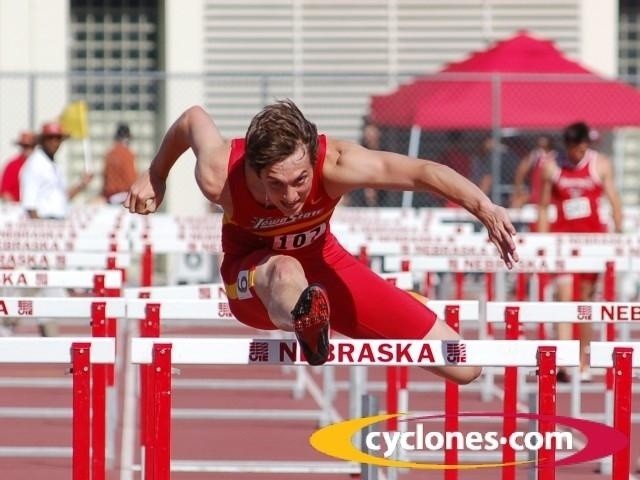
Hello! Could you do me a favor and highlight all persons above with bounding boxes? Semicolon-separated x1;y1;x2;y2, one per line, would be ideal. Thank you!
429;125;476;208
125;96;520;384
0;130;39;206
477;127;519;211
535;119;627;384
346;123;390;207
102;123;136;207
16;121;98;220
495;123;533;163
506;127;565;210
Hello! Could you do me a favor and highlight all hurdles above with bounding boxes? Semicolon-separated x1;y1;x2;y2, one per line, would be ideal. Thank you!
0;202;640;480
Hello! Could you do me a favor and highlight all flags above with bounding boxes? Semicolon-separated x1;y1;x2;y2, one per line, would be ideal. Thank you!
54;100;89;139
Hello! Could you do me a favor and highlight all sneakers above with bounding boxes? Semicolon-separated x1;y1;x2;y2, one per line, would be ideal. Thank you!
14;134;37;148
557;368;570;383
580;370;595;385
38;124;69;142
292;285;330;365
